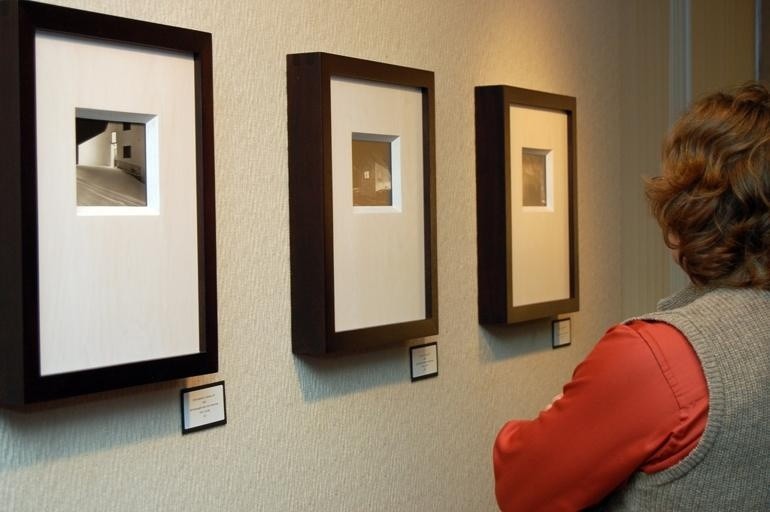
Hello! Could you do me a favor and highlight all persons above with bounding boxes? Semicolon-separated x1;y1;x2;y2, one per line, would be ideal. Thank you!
495;81;768;512
